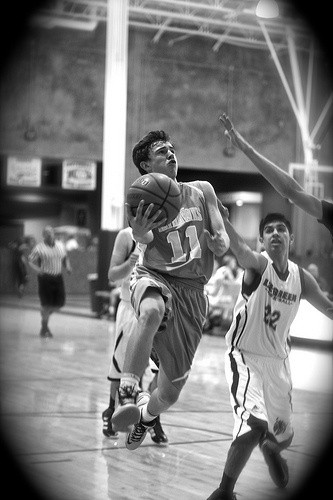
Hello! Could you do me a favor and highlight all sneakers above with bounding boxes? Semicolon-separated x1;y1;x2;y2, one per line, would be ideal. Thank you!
125;390;156;450
110;383;141;433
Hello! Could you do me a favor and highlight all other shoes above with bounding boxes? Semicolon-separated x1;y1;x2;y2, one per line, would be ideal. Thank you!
102;406;120;438
148;415;168;445
206;488;242;499
39;320;53;336
264;442;289;487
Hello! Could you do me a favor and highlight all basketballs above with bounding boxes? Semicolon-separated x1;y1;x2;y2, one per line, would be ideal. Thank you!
126;173;184;228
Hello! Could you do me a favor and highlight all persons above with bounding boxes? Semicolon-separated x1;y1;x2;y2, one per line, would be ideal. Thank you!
110;131;231;450
27;225;72;338
207;199;333;500
306;264;328;292
202;256;240;335
14;237;28;298
219;112;333;235
102;227;169;445
